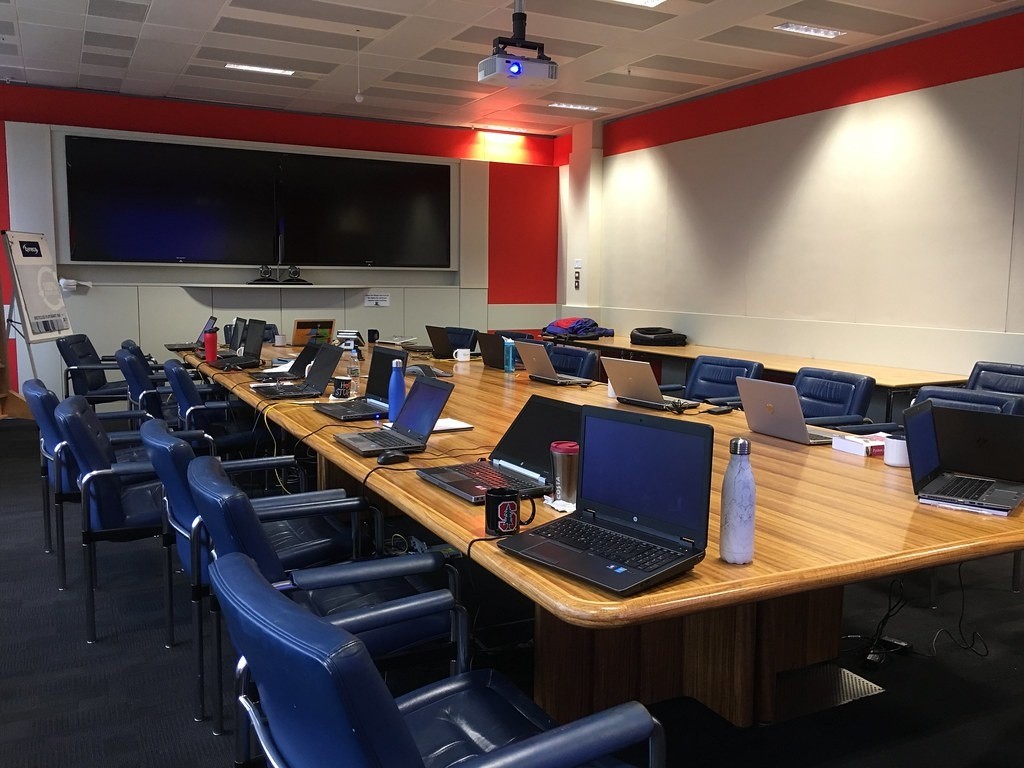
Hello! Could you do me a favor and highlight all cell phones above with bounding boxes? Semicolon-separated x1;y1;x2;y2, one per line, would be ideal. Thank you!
708;406;732;415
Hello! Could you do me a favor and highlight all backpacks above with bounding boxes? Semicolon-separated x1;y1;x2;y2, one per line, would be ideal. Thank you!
542;316;597;340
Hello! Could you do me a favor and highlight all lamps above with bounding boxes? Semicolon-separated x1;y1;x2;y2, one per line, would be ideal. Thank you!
355;31;363;103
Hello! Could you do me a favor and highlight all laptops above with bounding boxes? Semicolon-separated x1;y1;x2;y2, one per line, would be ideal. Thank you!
902;399;1024;509
195;316;714;599
735;377;845;446
164;316;217;351
932;405;1024;484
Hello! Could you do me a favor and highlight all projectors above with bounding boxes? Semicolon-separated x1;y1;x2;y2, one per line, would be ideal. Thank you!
478;54;559;87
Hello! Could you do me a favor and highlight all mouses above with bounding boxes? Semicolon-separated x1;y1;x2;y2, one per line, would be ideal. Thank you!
376;450;409;465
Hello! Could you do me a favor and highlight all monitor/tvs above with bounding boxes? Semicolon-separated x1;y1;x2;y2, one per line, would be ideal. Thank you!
64;134;452;268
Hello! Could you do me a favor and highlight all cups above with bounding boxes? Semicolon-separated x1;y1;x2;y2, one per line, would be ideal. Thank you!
884;436;910;468
368;329;379;343
485;488;536;536
608;379;617;397
334;376;356;398
237;347;244;357
550;441;579;504
305;361;315;378
276;335;286;346
453;349;470;361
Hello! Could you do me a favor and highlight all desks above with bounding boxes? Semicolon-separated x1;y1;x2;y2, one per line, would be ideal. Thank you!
176;343;1024;726
538;332;970;434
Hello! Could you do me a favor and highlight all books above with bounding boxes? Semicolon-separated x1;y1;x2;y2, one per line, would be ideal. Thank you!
382;418;474;433
832;432;892;456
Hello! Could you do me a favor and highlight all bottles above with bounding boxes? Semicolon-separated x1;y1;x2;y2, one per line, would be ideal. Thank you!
203;327;217;364
348;353;360;393
720;438;756;564
341;343;364;360
389;359;406;422
224;325;234;345
505;339;515;372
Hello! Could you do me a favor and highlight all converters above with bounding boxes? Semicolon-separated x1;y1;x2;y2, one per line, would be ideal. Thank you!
880;636;913;656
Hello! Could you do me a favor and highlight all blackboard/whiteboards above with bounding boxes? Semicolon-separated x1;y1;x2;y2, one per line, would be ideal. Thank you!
51;125;459;272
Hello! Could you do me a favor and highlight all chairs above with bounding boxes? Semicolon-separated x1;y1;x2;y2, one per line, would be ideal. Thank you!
965;360;1024;394
659;355;764;406
724;366;876;428
22;326;667;767
834;385;1024;440
